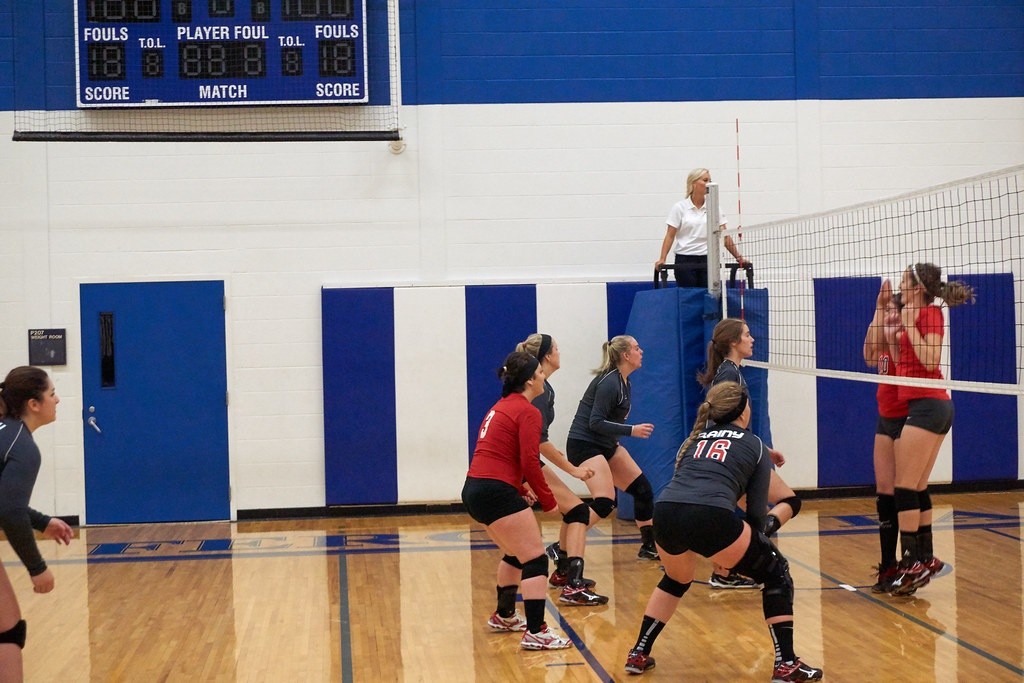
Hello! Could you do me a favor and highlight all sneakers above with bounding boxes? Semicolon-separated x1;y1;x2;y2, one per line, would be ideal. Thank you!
625;649;655;674
558;582;609;606
889;556;945;597
709;570;758;590
872;560;904;594
487;608;527;631
771;657;823;683
521;627;573;651
897;559;910;579
638;544;661;560
546;540;568;566
549;569;595;589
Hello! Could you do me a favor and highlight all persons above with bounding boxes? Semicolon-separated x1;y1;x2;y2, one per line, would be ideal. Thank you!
624;317;823;683
461;334;655;651
864;263;978;597
0;366;74;683
655;168;748;287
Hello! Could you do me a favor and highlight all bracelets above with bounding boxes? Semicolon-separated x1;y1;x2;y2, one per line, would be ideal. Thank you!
736;255;743;262
